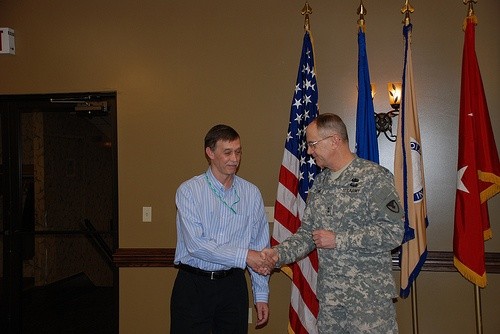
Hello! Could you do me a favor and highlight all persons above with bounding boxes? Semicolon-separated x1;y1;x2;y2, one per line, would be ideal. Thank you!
170;124;275;334
257;113;405;334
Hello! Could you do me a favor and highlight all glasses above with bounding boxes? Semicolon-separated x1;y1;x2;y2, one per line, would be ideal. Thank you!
307;136;341;150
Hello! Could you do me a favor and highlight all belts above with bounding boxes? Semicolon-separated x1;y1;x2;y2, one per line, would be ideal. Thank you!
178;263;240;280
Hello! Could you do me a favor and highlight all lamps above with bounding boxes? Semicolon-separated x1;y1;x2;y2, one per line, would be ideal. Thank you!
356;81;402;142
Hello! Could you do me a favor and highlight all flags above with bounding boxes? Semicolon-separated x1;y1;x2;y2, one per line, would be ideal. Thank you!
392;24;428;298
270;30;319;334
452;15;500;287
357;27;379;163
